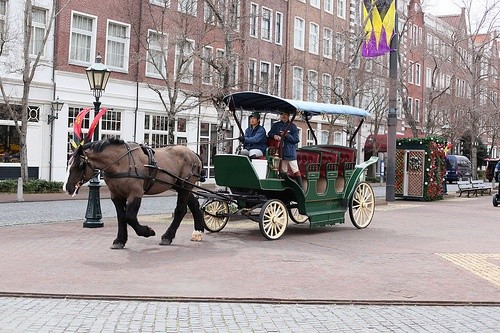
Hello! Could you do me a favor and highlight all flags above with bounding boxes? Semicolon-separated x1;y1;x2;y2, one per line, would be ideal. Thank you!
361;0;397;57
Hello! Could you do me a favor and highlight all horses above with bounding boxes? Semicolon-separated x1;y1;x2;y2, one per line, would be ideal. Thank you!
61;136;206;250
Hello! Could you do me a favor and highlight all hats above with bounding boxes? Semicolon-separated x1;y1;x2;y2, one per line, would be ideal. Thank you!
249;112;260;119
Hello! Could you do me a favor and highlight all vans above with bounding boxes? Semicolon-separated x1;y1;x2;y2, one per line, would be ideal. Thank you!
446;155;472;184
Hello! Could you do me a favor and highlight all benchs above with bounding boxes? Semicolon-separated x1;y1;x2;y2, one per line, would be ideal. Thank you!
321;144;358;176
315;148;341;179
287;148;323;179
457;180;484;198
255;136;285;159
471;179;494;196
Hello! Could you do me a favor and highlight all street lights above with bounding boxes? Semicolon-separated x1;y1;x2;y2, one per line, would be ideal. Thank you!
80;51;112;228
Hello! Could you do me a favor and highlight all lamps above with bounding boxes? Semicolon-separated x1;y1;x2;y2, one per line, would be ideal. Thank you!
47;95;65;124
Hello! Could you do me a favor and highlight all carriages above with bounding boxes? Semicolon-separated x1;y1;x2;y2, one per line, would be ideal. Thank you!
61;89;380;250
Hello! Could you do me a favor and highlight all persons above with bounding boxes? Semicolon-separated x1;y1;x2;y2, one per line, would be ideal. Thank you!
267;108;304;191
237;111;267;157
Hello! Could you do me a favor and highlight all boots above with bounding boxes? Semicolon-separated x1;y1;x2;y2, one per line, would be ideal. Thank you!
296;176;305;194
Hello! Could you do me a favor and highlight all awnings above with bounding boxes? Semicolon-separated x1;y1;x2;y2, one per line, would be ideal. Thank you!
364;134;425;152
